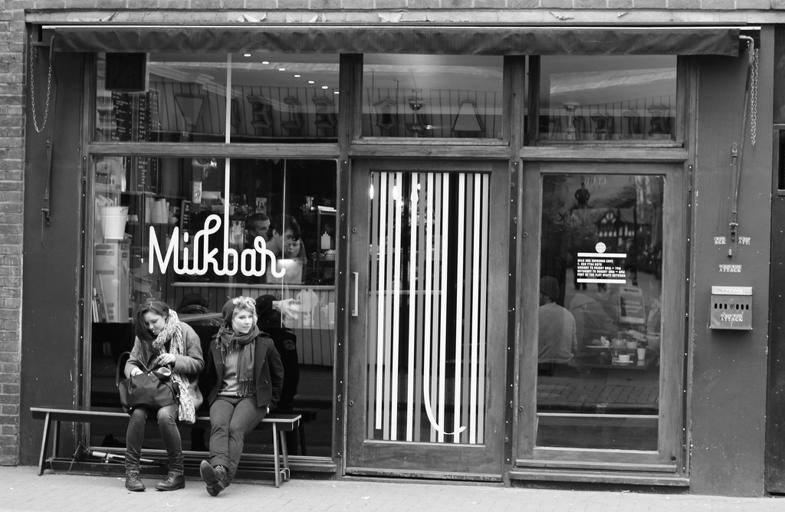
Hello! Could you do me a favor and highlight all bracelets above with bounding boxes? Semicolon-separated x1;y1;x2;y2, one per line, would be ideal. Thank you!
134;371;142;376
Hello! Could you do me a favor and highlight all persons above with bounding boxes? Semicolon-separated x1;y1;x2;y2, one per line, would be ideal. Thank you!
118;296;205;492
531;231;664;375
228;210;334;329
254;290;309;480
174;292;222;454
196;294;287;497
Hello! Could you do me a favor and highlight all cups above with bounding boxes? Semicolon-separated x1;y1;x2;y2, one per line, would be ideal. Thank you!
637;348;645;361
150;198;169;224
618;355;629;362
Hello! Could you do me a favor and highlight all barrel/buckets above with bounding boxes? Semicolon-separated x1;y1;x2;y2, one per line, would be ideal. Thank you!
98;205;129;241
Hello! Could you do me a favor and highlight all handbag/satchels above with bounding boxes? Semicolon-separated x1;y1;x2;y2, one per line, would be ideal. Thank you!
118;374;174;413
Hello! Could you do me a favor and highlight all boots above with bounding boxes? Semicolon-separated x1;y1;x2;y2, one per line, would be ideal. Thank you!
155;457;185;491
199;459;230;496
125;453;145;491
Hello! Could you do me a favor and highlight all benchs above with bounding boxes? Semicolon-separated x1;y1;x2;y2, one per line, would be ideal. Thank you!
29;403;303;489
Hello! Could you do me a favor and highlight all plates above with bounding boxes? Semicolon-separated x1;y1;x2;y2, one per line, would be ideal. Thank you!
615;360;634;365
